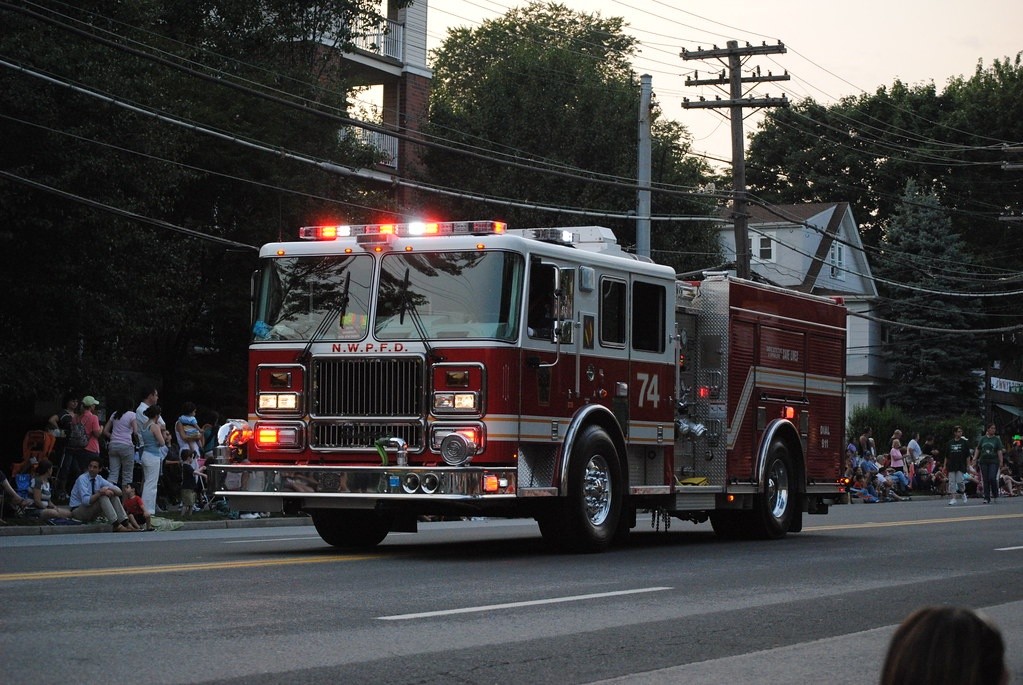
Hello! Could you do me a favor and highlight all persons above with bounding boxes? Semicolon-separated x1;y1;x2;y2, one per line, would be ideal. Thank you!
69;457;139;532
942;426;970;505
845;426;1023;502
0;386;274;522
141;405;167;530
971;422;1003;503
181;450;208;517
879;606;1009;685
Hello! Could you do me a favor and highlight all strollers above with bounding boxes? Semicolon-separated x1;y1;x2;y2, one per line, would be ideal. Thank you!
913;455;935;495
197;467;228;511
12;430;69;505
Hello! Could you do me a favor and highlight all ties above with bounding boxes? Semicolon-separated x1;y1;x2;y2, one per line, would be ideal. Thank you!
89;479;96;495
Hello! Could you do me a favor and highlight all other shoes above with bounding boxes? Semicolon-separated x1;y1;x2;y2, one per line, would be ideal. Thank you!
192;504;201;511
125;523;141;532
961;493;968;504
132;524;145;532
43;518;56;525
190;451;197;460
112;523;132;532
147;524;155;531
55;490;70;501
984;498;990;503
995;496;999;503
155;505;168;512
949;499;957;505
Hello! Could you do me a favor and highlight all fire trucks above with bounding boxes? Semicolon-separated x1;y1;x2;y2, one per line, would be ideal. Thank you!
209;219;851;557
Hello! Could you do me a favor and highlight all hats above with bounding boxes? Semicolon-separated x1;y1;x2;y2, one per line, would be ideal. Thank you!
81;396;100;406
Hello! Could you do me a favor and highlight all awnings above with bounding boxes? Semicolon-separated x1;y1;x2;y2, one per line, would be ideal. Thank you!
997;403;1023;428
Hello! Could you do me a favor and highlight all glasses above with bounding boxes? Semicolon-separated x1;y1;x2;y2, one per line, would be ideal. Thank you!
88;463;100;469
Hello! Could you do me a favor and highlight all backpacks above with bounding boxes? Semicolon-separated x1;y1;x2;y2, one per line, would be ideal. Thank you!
71;414;93;447
